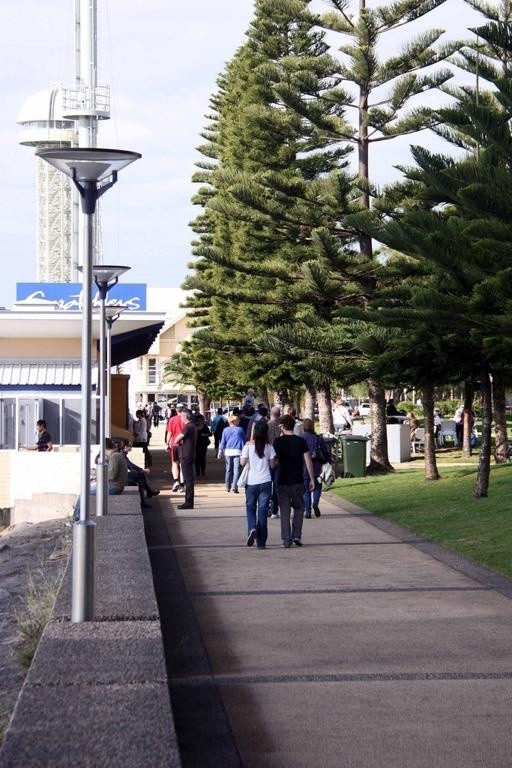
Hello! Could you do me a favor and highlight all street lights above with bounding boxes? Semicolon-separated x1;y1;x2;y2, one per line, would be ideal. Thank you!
34;147;143;635
101;301;128;440
70;254;133;521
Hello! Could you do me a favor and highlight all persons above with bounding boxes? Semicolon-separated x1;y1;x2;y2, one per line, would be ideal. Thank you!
36;420;53;451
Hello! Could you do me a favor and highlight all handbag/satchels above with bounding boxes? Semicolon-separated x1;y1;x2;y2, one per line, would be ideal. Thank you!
345;419;351;429
310;434;328;464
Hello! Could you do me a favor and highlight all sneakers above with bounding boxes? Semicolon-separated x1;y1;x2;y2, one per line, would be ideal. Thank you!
313;503;320;517
304;513;311;519
64;519;77;527
171;481;186;492
236;463;249;488
147;488;160;498
245;528;265;549
271;513;280;519
280;537;305;548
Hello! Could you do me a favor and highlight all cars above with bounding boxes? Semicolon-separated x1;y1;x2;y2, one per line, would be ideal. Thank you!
217;398;372;418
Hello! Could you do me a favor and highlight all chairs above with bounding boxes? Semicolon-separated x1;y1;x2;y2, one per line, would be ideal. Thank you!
437;419;458;449
411;426;425;454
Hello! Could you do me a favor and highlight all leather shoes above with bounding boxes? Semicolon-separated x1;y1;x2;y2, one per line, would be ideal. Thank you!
177;503;193;509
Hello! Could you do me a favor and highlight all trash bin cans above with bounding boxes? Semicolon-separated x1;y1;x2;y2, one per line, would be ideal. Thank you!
338;434;371;478
